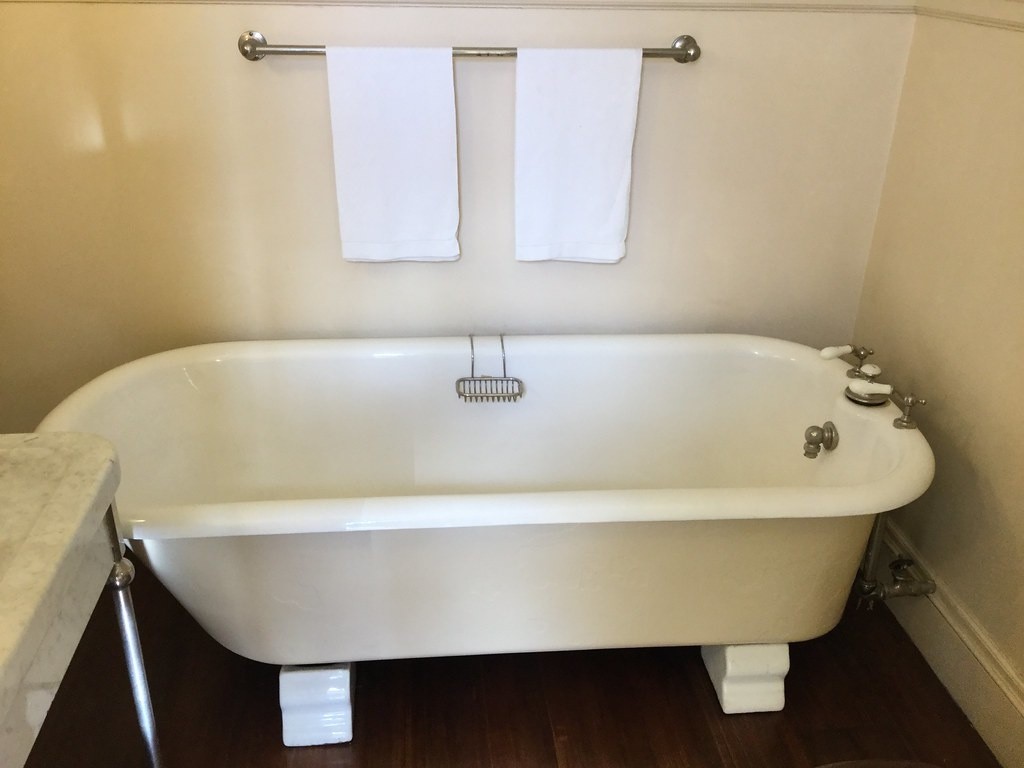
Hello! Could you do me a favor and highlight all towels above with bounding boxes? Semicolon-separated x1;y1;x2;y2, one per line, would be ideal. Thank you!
324;46;461;263
513;44;644;264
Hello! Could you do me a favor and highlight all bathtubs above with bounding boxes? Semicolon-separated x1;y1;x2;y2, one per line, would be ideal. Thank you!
29;335;938;669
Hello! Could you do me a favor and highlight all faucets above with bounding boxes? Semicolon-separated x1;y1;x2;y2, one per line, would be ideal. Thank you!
802;421;840;459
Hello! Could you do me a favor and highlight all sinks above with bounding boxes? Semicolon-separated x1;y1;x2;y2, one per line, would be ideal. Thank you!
0;431;124;767
